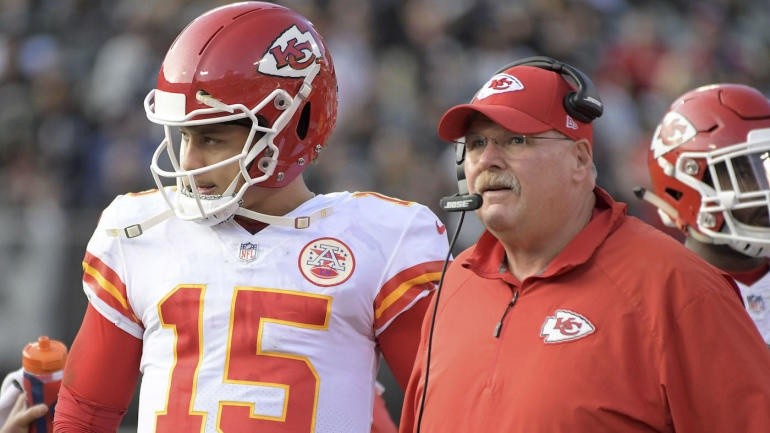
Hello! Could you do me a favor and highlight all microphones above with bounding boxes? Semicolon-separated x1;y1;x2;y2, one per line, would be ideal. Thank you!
439;194;483;212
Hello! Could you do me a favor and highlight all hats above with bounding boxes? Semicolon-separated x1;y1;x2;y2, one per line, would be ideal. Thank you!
438;65;593;148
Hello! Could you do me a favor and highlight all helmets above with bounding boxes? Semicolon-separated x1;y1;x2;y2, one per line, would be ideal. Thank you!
648;84;770;235
153;2;337;188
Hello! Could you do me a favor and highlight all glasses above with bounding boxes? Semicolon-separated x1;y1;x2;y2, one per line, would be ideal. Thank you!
454;135;571;157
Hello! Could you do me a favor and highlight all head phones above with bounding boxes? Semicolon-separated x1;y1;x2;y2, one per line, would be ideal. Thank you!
455;56;604;195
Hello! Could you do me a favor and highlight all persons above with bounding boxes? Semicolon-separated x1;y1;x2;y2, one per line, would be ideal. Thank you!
396;56;770;433
0;392;48;433
52;2;455;433
630;83;770;344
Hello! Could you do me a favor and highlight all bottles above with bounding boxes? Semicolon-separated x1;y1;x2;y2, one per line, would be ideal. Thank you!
23;335;70;430
